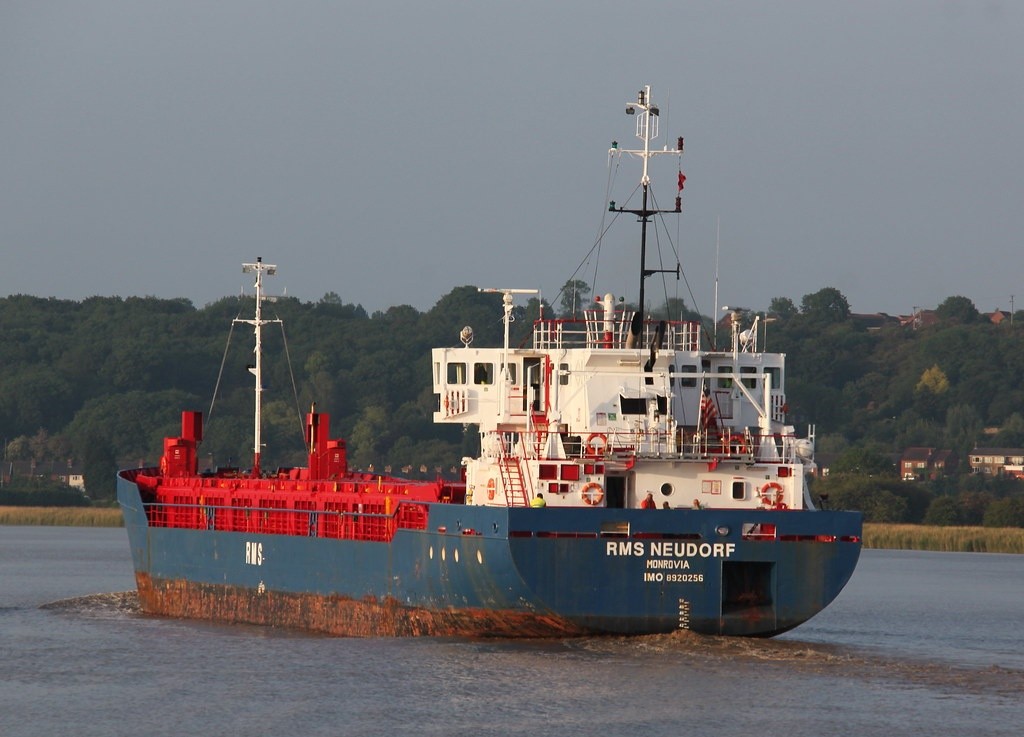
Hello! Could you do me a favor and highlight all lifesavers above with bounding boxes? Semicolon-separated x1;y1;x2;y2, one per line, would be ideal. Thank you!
762;482;784;506
581;482;604;505
725;435;746;458
586;433;608;455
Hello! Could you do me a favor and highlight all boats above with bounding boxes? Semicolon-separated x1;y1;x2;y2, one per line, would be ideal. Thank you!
112;81;866;638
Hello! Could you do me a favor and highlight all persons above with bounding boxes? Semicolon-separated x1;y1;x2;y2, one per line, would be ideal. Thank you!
641;494;704;510
529;493;546;508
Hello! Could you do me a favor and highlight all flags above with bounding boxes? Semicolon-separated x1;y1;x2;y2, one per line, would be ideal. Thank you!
701;381;716;427
